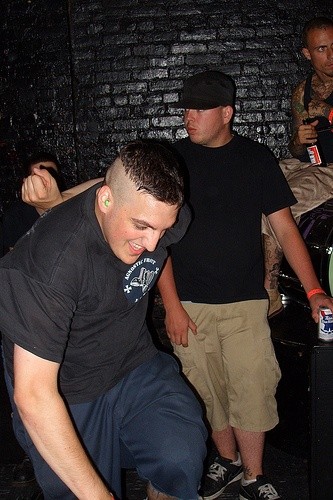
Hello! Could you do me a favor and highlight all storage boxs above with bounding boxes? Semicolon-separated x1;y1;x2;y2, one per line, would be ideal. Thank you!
308;325;333;500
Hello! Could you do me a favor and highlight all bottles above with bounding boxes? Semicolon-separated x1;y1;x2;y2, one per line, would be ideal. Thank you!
302;119;323;167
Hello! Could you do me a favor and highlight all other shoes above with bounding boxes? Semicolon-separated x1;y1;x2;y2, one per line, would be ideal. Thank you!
266;290;285;320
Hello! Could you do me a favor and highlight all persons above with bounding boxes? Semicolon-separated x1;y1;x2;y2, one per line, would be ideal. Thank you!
156;71;333;500
0;138;208;500
262;17;333;318
0;154;105;487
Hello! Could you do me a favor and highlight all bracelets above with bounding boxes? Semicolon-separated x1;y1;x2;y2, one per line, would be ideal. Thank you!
307;288;327;300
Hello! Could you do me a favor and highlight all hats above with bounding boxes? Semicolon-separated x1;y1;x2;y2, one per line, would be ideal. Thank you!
169;70;236;110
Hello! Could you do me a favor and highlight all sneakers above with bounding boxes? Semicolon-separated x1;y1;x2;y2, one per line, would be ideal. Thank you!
196;452;244;500
239;474;284;500
